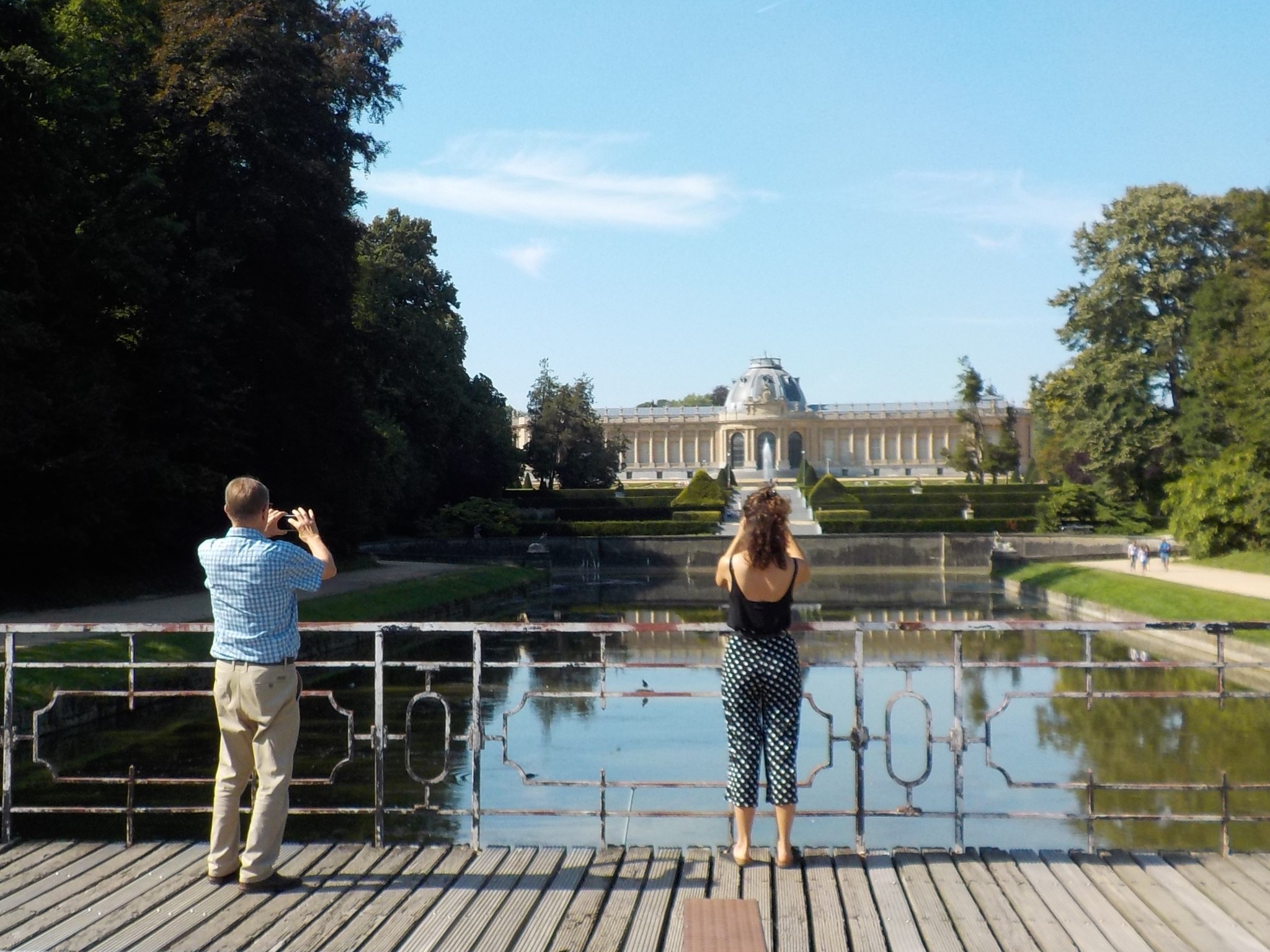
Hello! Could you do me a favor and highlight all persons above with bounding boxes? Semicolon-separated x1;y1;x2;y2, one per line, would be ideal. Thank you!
1128;540;1139;574
198;477;336;893
715;487;813;869
1157;537;1171;573
1138;544;1151;572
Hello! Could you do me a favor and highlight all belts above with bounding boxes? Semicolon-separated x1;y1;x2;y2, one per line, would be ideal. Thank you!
276;657;295;666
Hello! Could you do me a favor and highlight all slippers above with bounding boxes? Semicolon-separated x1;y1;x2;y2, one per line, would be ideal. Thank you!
775;846;801;869
719;842;752;867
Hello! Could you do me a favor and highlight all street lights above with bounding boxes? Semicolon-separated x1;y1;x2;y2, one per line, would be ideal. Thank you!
727;452;732;488
801;449;806;485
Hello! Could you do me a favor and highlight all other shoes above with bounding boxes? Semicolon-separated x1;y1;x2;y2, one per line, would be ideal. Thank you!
239;872;303;893
207;863;242;884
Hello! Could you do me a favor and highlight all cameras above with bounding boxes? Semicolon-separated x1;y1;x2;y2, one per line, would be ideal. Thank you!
278;515;298;531
